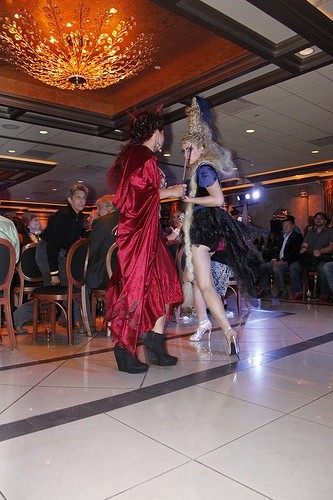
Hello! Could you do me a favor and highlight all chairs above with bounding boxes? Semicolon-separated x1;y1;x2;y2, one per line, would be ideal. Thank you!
0;238;242;351
302;271;319;301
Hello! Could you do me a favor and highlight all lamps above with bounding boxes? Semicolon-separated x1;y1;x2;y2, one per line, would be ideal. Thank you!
0;0;160;90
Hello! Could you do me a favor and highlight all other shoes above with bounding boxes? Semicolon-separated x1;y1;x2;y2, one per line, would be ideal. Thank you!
327;295;333;302
15;326;28;334
275;290;303;300
256;289;272;298
57;319;67;327
80;326;96;334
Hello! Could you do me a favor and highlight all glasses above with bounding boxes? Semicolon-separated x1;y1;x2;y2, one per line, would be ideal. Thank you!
75;196;87;200
314;217;325;220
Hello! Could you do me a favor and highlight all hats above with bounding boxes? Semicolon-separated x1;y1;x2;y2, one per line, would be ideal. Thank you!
280;215;295;224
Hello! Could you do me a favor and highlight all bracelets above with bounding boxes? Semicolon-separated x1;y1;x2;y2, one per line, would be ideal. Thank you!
194;197;197;205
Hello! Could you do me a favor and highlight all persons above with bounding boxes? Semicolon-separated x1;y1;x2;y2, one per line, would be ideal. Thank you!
108;112;187;374
248;209;333;304
181;97;239;356
0;183;193;337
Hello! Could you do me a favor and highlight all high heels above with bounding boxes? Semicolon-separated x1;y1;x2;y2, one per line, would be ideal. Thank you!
144;329;178;367
113;343;148;374
223;327;240;360
188;319;213;341
